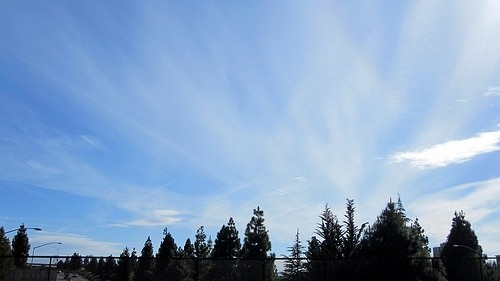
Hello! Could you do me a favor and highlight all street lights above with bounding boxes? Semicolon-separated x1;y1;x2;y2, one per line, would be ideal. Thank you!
453;242;484;280
32;241;62;262
